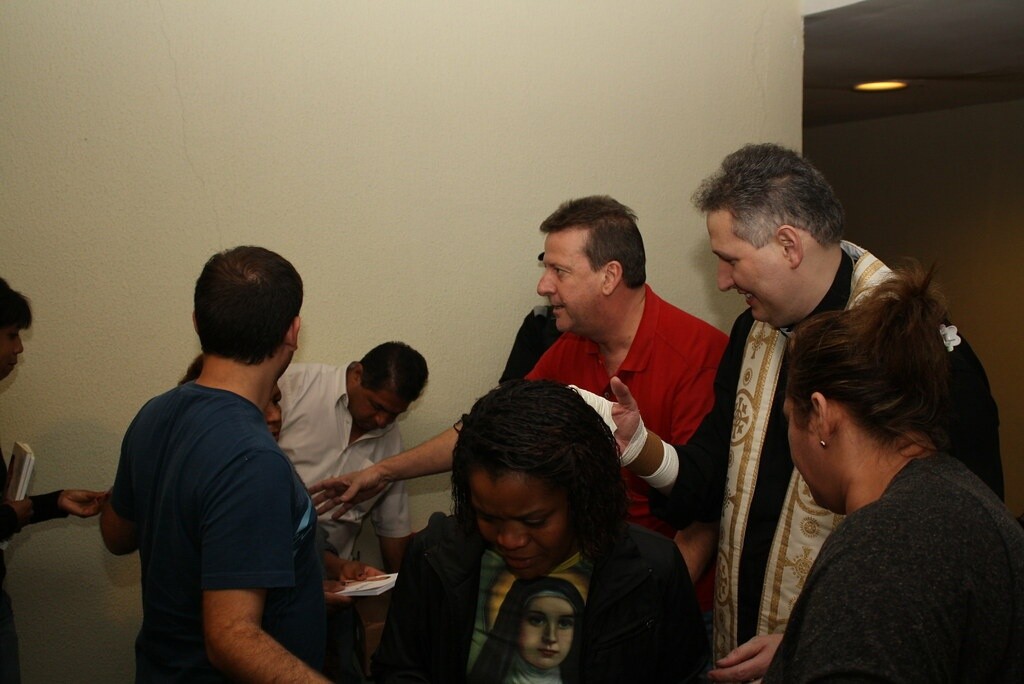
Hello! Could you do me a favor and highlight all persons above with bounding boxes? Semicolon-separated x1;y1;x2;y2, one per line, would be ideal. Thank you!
498;253;562;386
344;576;390;586
369;378;705;684
4;455;15;505
305;194;731;684
0;277;107;684
176;354;387;684
278;341;428;684
568;142;1005;684
101;245;330;684
761;262;1024;684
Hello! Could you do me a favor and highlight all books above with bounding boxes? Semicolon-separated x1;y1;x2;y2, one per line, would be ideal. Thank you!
0;442;35;549
335;573;398;596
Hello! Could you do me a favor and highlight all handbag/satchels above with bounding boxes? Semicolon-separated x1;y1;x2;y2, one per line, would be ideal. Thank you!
320;604;367;684
